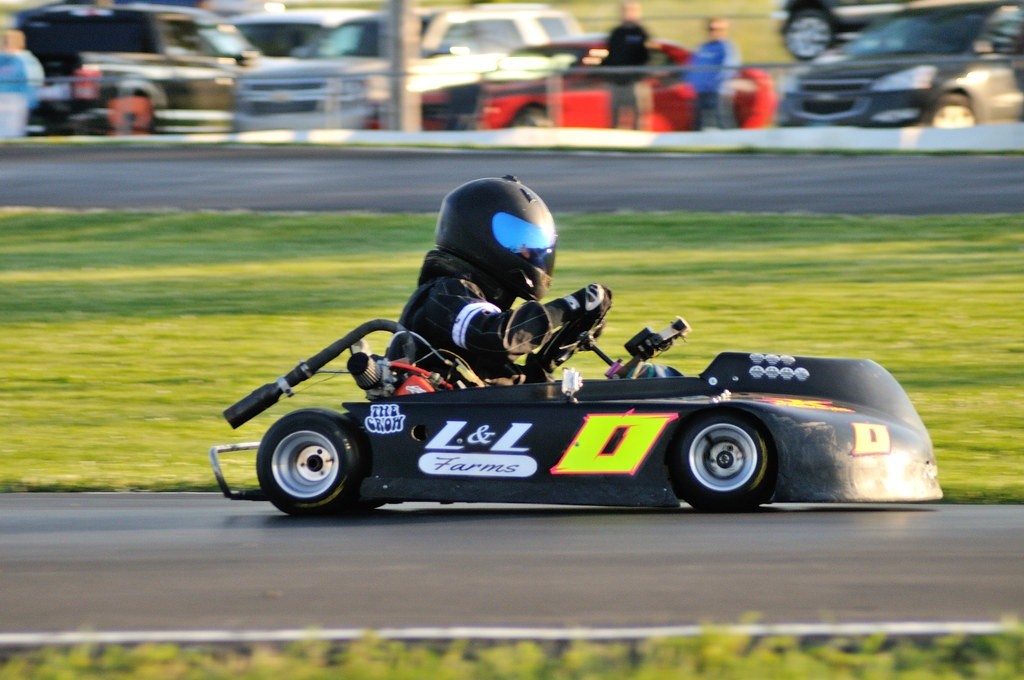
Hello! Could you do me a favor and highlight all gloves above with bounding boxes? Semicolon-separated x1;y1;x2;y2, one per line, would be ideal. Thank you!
557;283;611;322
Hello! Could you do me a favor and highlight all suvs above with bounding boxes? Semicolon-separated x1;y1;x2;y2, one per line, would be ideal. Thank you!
12;0;582;134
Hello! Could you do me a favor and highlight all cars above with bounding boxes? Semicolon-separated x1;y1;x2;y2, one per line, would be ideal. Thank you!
362;34;779;132
777;0;1024;127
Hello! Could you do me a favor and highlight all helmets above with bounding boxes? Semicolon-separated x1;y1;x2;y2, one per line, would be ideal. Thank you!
435;174;557;301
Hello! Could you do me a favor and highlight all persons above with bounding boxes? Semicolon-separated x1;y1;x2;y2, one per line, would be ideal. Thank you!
597;0;652;130
684;15;738;130
392;174;685;387
0;29;44;138
